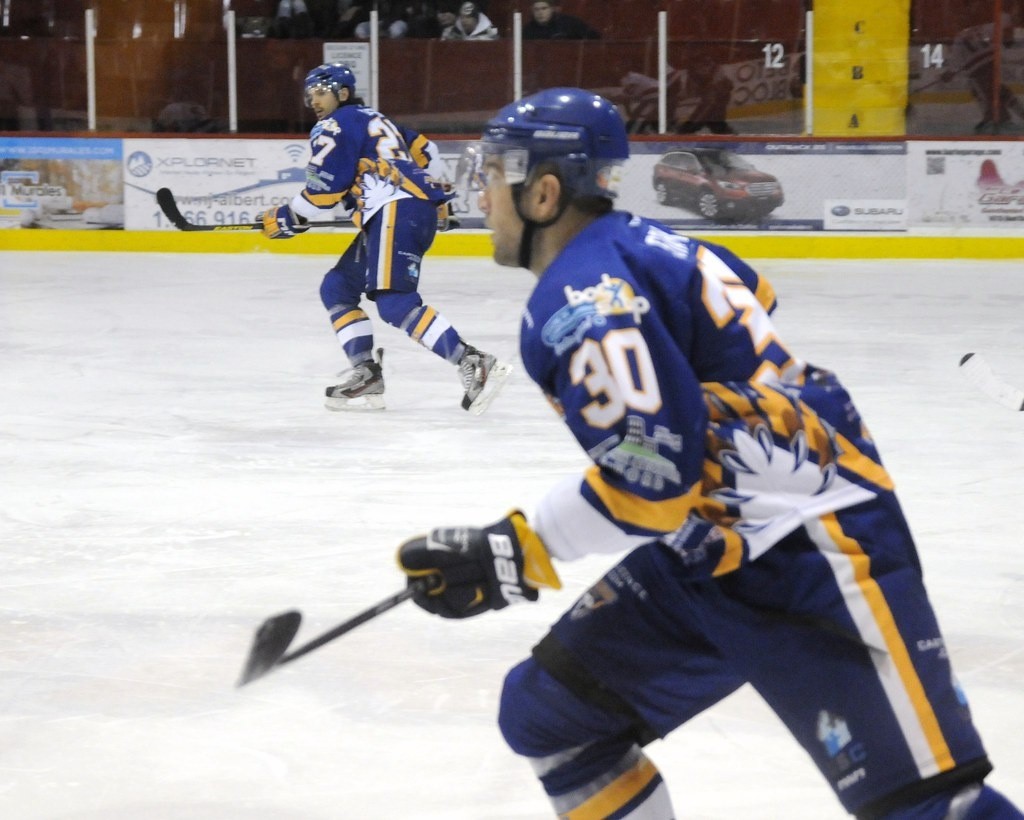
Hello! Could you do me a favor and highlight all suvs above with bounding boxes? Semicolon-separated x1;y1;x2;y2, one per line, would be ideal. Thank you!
653;150;785;222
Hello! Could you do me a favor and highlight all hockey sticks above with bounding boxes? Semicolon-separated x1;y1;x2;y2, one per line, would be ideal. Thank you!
953;349;1024;413
155;186;356;235
234;573;443;690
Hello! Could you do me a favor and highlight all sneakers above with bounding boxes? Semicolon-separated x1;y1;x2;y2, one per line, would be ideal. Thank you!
324;348;386;411
458;344;515;415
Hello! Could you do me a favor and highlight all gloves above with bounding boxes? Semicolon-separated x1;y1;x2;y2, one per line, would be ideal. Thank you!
258;204;311;240
435;203;464;231
396;506;561;621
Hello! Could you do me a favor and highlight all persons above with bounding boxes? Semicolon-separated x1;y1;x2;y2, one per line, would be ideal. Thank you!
253;62;496;413
239;2;602;52
399;85;1024;820
937;16;1021;134
603;34;733;143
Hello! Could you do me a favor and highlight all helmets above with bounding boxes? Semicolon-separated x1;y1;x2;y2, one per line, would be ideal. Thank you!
480;87;630;197
304;63;356;98
